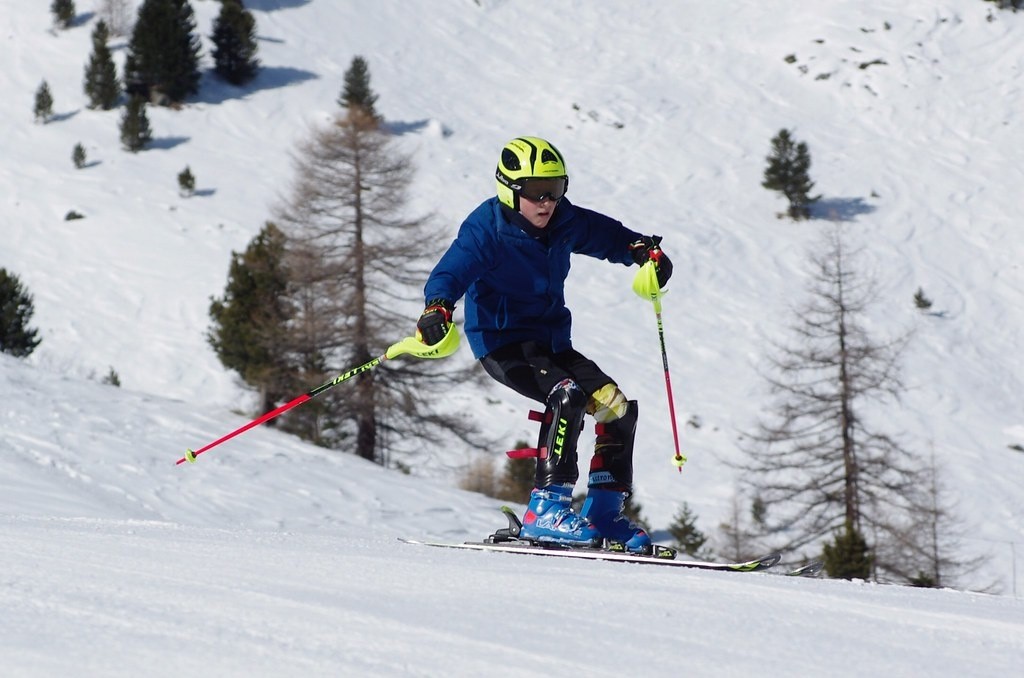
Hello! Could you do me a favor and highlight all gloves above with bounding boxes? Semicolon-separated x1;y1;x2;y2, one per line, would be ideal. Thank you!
628;235;673;289
416;299;457;346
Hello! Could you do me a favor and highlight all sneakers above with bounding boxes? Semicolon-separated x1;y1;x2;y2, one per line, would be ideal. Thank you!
520;482;602;547
579;487;651;553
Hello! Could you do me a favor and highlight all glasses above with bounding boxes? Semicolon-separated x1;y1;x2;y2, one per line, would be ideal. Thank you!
495;166;568;202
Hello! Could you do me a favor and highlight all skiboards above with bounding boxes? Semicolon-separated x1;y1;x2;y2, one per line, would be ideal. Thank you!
394;537;826;579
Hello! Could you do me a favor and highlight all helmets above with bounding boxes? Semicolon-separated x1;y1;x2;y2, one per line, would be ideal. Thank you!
496;136;566;214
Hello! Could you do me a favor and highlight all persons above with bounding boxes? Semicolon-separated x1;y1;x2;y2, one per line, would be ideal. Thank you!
415;139;674;553
